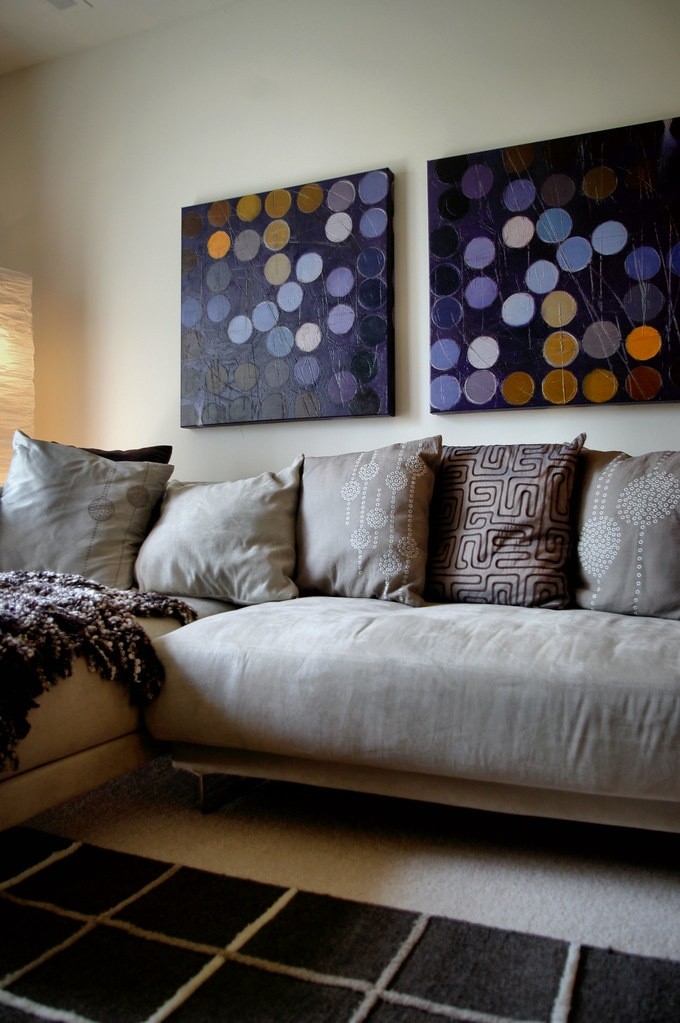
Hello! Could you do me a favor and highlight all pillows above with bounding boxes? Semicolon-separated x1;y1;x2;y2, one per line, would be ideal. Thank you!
0;428;176;590
432;431;589;610
51;440;174;464
135;454;306;604
298;432;443;608
561;441;680;620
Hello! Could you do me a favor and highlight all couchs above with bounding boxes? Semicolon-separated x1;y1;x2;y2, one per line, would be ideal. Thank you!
1;482;680;827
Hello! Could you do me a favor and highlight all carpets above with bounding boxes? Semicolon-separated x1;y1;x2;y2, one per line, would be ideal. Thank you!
0;826;679;1023
